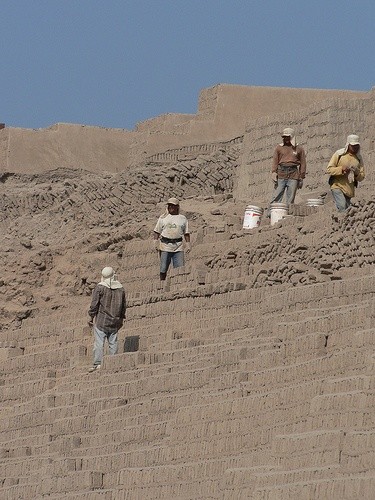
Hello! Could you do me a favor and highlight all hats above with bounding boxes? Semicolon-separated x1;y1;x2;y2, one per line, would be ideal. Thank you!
278;128;298;149
97;266;123;290
165;198;180;207
337;135;361;161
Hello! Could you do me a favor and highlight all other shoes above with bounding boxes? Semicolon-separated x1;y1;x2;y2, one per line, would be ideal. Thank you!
88;364;101;373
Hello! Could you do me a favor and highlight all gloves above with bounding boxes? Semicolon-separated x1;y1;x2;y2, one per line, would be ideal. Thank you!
185;242;192;254
86;314;94;328
347;170;354;184
271;172;278;184
298;178;303;190
350;166;360;175
154;240;160;251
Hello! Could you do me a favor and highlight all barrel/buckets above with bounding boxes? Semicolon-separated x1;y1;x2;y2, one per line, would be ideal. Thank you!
269;202;289;226
243;204;264;230
306;198;324;207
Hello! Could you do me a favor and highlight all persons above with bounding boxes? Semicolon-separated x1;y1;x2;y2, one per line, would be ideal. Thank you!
153;198;191;280
325;134;366;212
266;127;307;218
88;266;127;374
